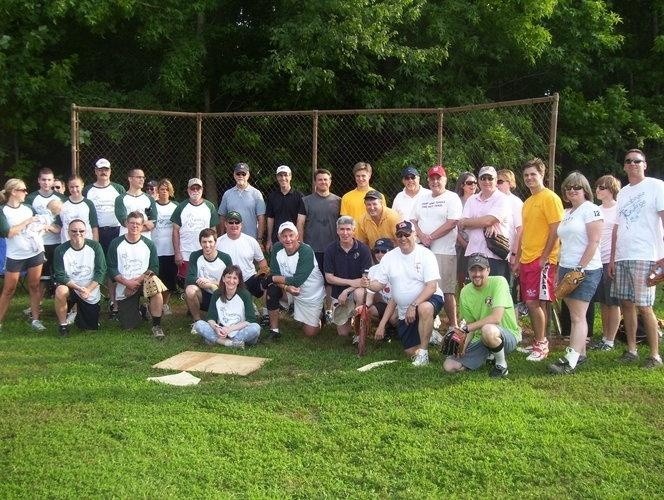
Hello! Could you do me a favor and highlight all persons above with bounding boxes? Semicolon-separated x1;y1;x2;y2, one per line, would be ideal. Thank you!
547;149;664;373
107;169;219;338
0;159;127;335
184;159;343;342
326;158;564;377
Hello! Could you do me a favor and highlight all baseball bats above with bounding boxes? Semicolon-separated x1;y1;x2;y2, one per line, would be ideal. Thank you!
359;270;369;355
552;298;561;335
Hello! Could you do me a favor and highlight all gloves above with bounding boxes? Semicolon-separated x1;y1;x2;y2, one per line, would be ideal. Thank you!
440;327;467;357
262;275;275;289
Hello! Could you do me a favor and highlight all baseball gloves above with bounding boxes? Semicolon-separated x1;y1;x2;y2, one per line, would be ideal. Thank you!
647;258;664;287
512;272;524;304
553;268;585;299
254;266;270;279
484;231;510;259
441;326;467;355
143;270;169;298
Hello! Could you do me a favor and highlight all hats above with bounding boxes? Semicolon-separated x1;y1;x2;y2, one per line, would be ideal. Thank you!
364;190;382;199
234;163;250;172
95;158;111;170
374;238;394;249
400;167;419;176
279;221;297;233
226;211;242;223
479;166;498;179
428;166;446;177
276;166;292;176
396;222;415;234
188;178;203;188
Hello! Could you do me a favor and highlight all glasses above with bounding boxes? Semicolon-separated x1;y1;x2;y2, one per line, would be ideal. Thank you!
147;187;155;191
498;179;508;185
190;187;201;191
623;158;644;164
227;221;240;225
564;184;584;191
396;233;411;238
374;249;388;254
70;229;85;234
235;172;247;176
51;186;61;190
15;188;27;193
464;180;478;186
404;175;417;180
129;176;146;180
594;185;608;190
481;176;493;181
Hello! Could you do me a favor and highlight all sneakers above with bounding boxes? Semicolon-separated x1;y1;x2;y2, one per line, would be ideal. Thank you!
642;356;663;370
30;320;48;332
23;305;43;315
430;330;443;346
517;337;550;361
139;303;172;341
411;350;430;367
612;348;639;365
352;334;360;346
548;354;588;372
108;299;118;316
324;310;334;325
58;310;80;338
233;341;245;351
486;352;509;378
259;314;281;340
588;339;615;352
